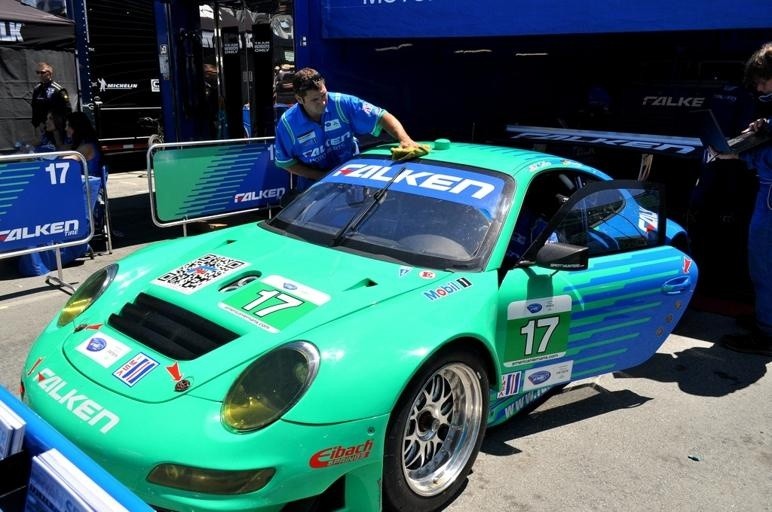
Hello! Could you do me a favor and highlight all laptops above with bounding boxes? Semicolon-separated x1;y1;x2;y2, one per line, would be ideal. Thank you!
689;108;769;155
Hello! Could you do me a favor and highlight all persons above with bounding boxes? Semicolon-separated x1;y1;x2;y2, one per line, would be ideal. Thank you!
273;65;423;194
7;61;107;277
449;187;560;286
707;44;772;357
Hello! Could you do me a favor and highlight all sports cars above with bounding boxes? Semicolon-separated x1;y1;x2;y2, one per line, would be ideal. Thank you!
18;122;711;512
